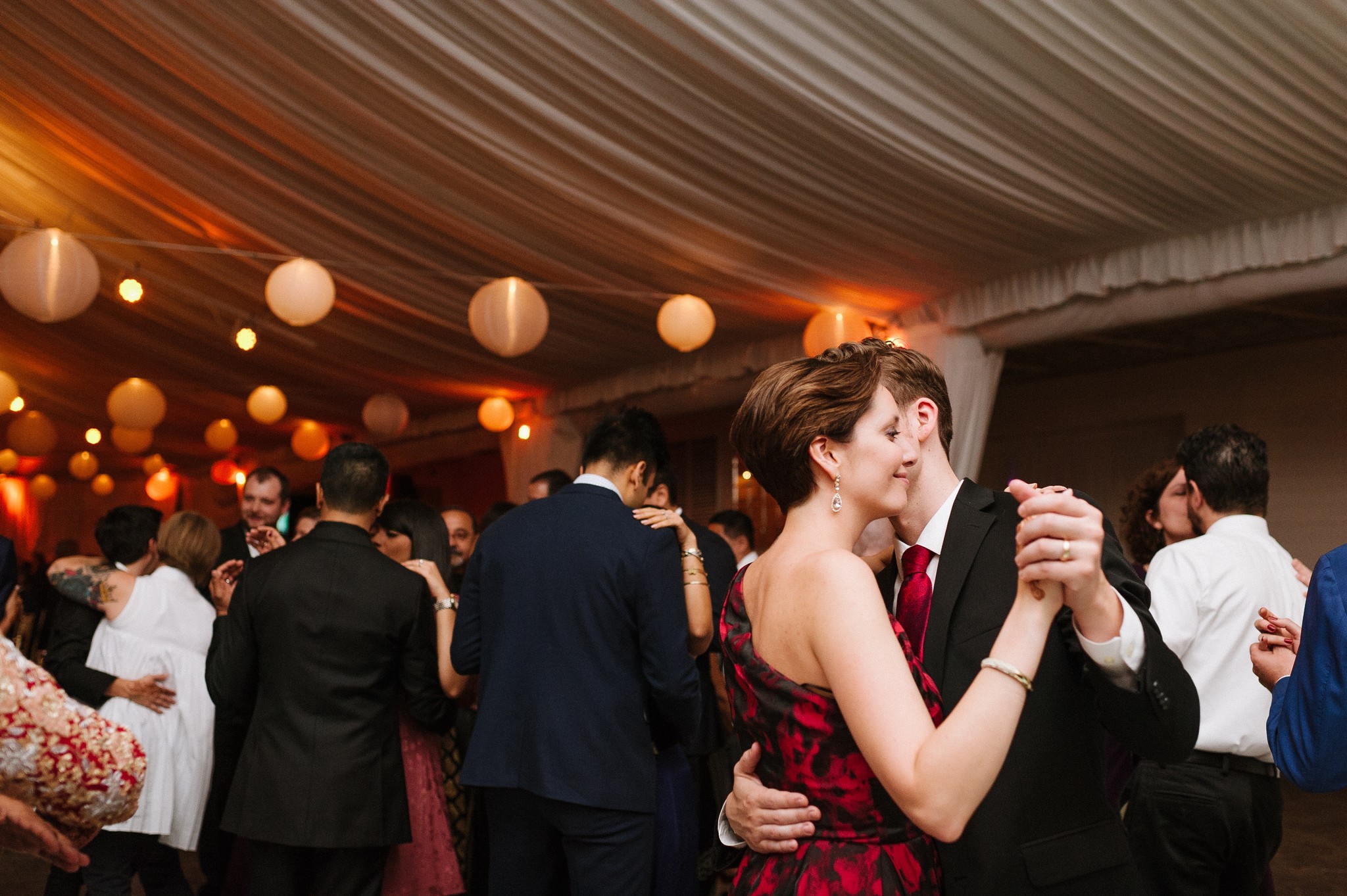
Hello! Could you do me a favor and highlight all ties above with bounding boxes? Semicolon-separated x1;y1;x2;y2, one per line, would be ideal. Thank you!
895;545;937;665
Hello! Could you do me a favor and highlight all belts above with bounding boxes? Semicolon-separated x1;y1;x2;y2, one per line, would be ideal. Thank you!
1185;749;1284;778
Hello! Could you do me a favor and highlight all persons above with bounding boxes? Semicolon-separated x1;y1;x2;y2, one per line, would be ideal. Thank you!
622;504;715;896
42;503;177;894
698;508;762;570
206;443;460;896
1099;450;1312;895
714;338;1200;896
242;498;473;896
449;407;706;896
45;511;220;895
639;459;738;856
287;467;576;593
1;528;146;873
717;340;1073;896
193;465;289;610
1125;427;1310;896
1250;540;1347;794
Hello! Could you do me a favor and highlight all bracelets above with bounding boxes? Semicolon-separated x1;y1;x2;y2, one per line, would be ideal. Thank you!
979;658;1034;698
679;548;711;588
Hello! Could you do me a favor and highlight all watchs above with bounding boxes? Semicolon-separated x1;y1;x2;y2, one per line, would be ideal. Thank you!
433;592;460;615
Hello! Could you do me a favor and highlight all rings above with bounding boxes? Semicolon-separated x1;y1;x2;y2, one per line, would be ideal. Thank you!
1058;539;1070;562
661;512;669;520
418;559;423;567
225;577;233;585
